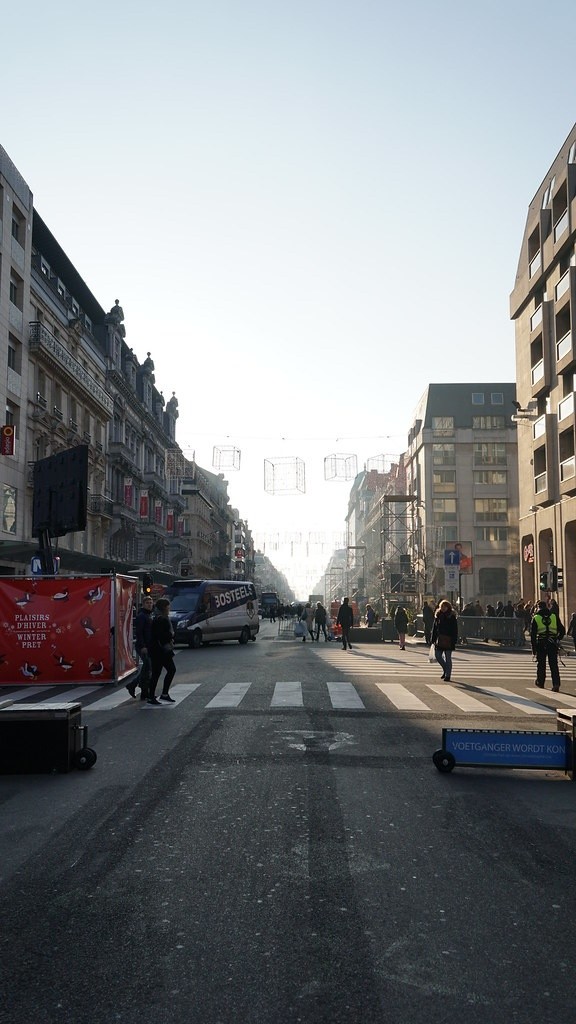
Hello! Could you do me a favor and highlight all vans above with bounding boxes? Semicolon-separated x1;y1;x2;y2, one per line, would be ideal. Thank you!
160;579;259;648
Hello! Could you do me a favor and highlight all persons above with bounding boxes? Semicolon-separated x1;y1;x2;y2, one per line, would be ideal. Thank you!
270;605;276;623
455;543;467;559
422;602;434;644
567;613;576;651
463;599;540;643
125;596;177;706
530;600;565;691
336;596;353;650
394;606;409;651
278;604;303;620
549;599;558;613
315;602;328;642
365;604;375;628
300;603;314;642
430;600;458;681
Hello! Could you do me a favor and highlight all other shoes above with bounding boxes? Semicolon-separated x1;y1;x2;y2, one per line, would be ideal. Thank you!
535;680;544;688
441;672;445;678
444;677;450;681
349;644;352;649
342;647;346;650
126;682;137;698
140;694;157;700
550;686;558;692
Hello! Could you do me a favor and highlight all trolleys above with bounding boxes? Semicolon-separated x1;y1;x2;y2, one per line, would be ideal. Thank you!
327;626;341;641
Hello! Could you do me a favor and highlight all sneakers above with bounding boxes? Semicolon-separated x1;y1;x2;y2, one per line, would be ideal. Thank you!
159;695;175;703
146;698;161;705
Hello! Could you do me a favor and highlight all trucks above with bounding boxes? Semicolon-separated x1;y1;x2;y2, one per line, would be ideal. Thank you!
309;595;324;608
260;592;280;617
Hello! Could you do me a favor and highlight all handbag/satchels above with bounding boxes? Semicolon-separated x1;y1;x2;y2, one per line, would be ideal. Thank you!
301;608;307;620
294;621;307;637
428;643;437;663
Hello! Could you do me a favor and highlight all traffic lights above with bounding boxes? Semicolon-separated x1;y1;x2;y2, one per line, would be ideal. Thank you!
557;567;564;591
539;571;548;590
